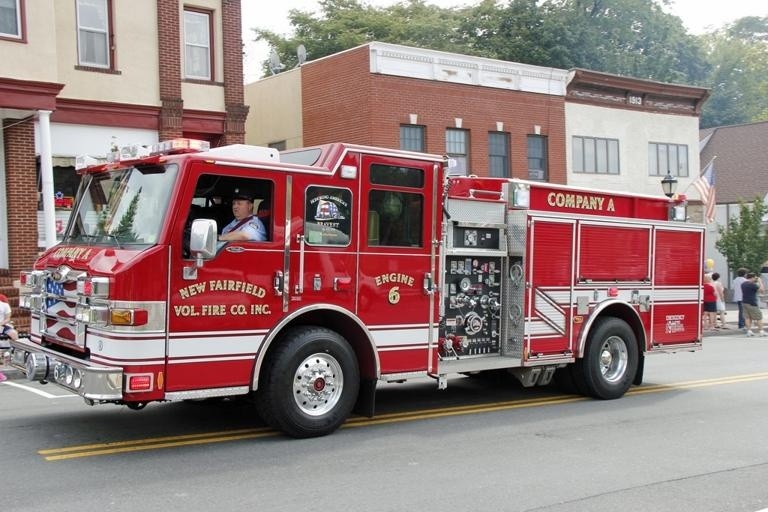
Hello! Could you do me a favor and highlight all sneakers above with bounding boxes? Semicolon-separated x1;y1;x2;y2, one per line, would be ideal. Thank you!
702;325;767;337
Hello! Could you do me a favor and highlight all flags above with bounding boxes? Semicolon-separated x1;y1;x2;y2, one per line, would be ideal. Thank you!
693;160;716;224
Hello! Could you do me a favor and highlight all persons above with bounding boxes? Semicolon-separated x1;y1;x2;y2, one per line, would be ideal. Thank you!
731;268;748;329
703;274;720;329
214;186;267;242
740;273;768;336
0;302;19;358
710;273;729;329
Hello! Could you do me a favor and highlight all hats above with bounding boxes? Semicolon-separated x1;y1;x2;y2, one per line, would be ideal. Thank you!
232;188;254;202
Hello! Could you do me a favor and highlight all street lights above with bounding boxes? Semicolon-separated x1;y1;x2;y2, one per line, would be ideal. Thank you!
659;169;678;221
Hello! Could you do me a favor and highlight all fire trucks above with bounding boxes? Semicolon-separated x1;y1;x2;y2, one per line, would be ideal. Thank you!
10;137;709;437
